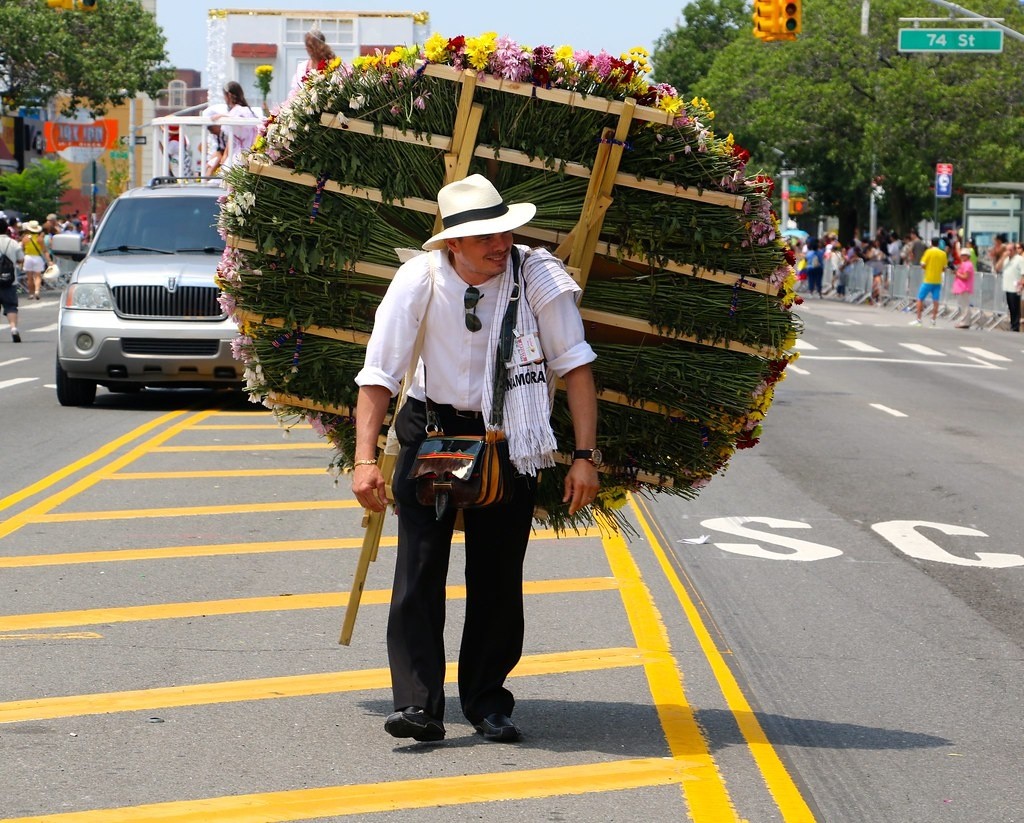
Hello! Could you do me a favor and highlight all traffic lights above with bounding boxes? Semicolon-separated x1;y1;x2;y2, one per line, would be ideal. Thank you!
781;0;801;34
757;0;780;34
793;198;808;213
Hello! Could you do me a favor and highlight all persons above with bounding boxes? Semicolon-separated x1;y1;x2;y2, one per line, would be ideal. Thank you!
160;81;270;180
352;174;600;742
293;31;344;91
0;219;25;342
794;228;1024;331
0;211;98;300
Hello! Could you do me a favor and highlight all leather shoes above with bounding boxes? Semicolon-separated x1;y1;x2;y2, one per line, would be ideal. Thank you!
473;711;521;740
384;707;446;742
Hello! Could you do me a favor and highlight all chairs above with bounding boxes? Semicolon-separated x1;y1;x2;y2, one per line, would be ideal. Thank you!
141;226;172;250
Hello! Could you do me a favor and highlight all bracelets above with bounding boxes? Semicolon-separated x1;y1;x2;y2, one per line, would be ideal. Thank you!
353;460;377;467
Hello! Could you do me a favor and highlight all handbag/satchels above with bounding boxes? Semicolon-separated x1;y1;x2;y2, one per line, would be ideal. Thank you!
42;264;60;279
404;430;513;521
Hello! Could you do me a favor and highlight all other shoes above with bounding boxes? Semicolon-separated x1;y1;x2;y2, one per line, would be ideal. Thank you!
35;290;40;300
955;323;970;328
909;319;923;326
930;319;936;325
11;328;21;341
28;295;33;299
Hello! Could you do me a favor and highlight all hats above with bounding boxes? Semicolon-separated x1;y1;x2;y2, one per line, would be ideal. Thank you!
160;113;179;134
422;173;537;252
22;219;42;232
960;247;970;254
828;231;836;238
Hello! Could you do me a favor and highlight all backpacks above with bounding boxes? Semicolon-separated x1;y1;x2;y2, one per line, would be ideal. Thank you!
0;237;15;285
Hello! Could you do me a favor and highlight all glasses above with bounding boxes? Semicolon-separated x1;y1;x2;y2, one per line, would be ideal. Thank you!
463;284;484;332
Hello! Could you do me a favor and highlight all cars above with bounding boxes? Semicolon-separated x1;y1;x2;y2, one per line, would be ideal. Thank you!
49;176;256;409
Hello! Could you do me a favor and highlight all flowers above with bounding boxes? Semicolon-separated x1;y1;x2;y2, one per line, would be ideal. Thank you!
208;31;806;543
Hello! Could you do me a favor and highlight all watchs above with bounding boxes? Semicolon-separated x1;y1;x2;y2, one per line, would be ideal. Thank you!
571;449;603;468
